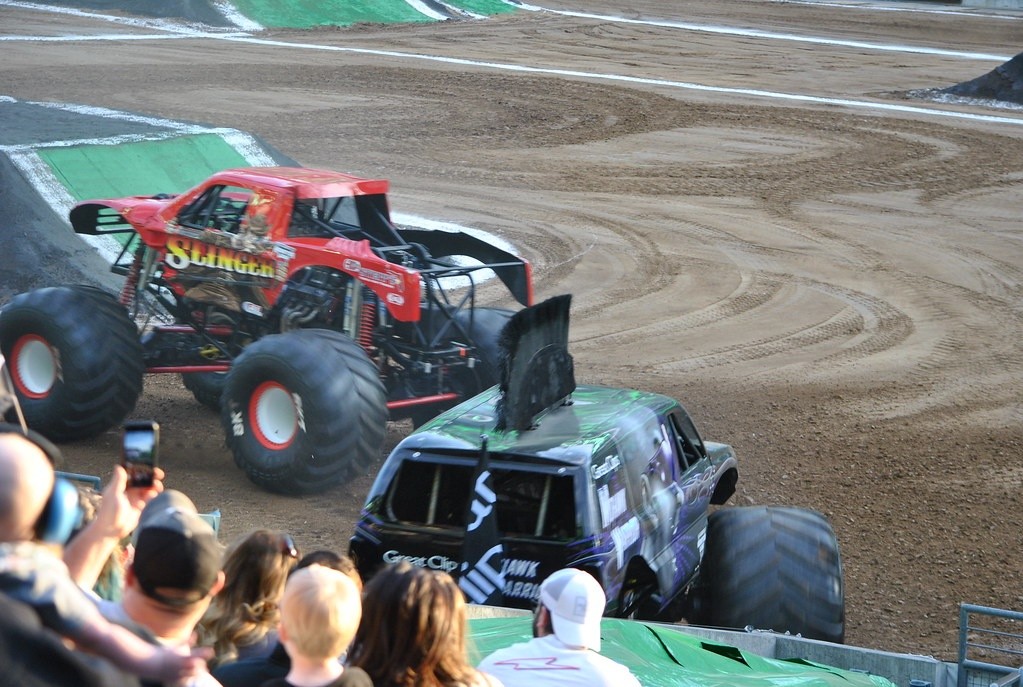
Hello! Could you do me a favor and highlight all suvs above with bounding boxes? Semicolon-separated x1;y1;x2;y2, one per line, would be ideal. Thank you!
0;165;535;498
347;294;849;642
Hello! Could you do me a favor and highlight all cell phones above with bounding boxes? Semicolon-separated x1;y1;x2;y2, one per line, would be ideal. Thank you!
121;420;159;489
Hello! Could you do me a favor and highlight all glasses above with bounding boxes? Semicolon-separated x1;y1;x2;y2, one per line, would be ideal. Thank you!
280;532;299;559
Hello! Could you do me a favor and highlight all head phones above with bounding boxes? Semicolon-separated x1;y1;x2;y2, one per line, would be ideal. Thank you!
0;422;84;546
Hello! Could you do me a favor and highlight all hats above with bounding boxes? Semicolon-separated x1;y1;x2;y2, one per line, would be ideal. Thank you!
540;568;605;652
131;489;224;606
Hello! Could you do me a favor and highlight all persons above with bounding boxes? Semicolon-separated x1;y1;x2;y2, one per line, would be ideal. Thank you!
0;433;502;687
475;568;642;687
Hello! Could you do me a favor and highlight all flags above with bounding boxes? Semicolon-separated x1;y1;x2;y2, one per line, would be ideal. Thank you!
457;442;504;605
